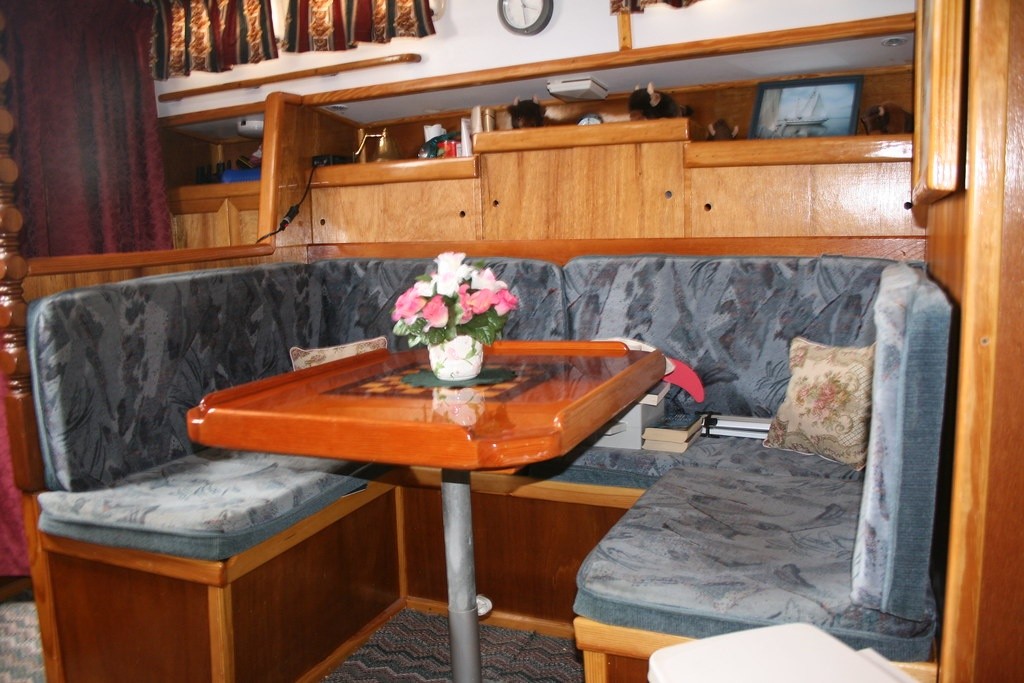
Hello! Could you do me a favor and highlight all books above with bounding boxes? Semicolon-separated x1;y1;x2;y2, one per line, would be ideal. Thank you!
639;381;670;406
641;411;771;454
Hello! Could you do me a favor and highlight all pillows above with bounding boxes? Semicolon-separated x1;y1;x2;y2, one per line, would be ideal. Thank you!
290;335;388;372
763;336;881;472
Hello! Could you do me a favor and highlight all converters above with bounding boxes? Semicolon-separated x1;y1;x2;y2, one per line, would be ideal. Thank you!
312;154;355;167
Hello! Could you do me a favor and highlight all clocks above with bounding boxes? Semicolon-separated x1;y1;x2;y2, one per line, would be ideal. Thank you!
497;0;553;37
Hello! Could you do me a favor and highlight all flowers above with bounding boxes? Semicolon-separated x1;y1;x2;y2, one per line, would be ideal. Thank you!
388;251;520;348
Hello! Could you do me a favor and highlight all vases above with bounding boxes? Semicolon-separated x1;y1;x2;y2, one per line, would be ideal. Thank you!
431;387;483;427
427;334;483;382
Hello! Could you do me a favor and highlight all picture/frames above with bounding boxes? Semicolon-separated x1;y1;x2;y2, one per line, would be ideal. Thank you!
747;74;865;140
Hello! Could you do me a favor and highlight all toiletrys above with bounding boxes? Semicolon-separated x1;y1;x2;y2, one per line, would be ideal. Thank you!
459;103;494;155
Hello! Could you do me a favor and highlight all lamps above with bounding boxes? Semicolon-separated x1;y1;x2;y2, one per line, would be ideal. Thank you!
236;121;265;139
546;76;609;104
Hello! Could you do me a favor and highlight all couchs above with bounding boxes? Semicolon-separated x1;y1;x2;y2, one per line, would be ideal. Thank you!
24;256;954;683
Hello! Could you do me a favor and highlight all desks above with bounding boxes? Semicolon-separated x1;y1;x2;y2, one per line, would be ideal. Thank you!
187;340;667;683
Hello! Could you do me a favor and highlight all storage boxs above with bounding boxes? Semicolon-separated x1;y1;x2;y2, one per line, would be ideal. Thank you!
592;398;665;449
